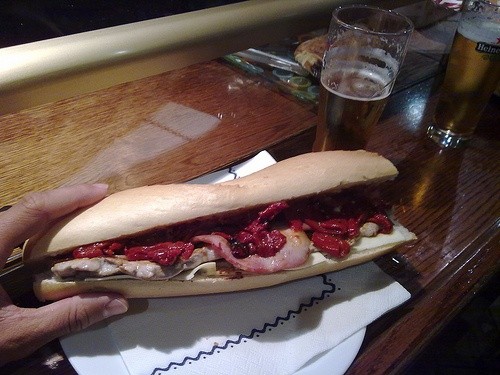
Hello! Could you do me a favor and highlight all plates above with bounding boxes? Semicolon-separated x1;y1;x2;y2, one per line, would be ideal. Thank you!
56;325;424;375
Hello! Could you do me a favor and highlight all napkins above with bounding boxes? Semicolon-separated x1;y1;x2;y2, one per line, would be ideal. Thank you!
50;149;411;375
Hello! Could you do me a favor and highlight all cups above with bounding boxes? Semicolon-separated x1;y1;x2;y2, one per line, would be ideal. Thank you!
426;0;500;153
312;4;414;152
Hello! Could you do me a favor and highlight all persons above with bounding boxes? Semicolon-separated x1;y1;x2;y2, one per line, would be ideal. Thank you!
0;183;128;367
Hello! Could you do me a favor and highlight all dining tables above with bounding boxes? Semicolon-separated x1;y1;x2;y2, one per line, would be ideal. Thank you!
0;12;500;375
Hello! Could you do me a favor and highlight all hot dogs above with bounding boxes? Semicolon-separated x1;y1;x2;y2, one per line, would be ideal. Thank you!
23;149;419;301
